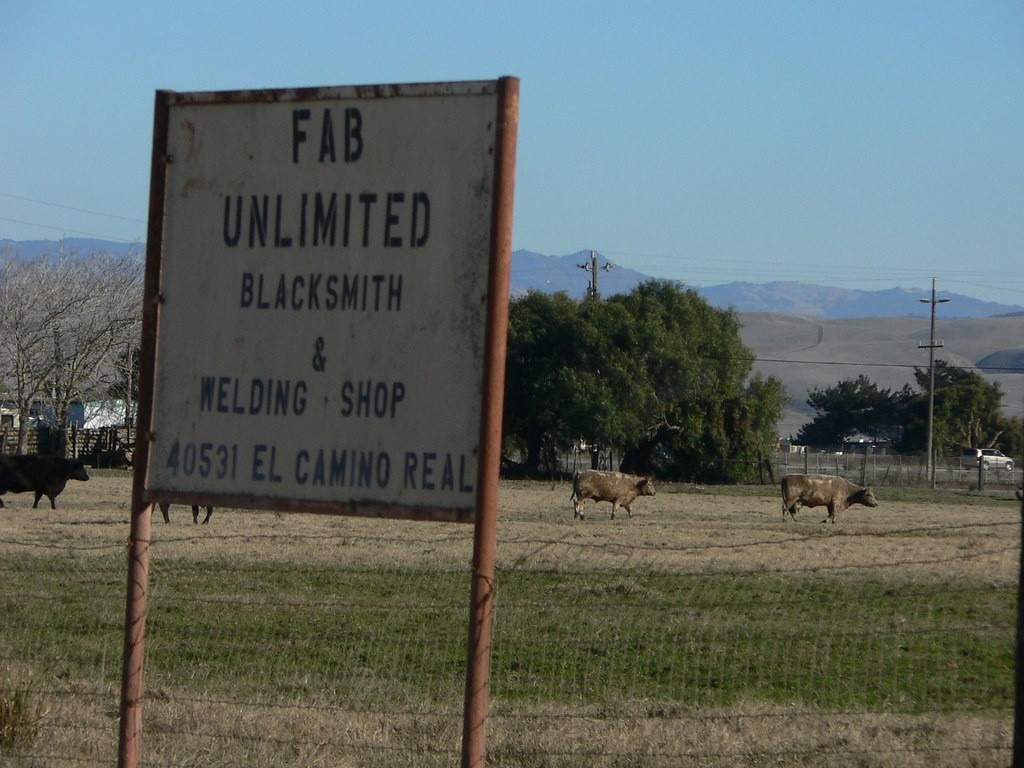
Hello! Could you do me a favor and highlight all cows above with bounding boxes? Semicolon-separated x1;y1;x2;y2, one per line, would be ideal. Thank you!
780;474;878;524
151;503;213;525
570;469;656;520
0;454;90;509
